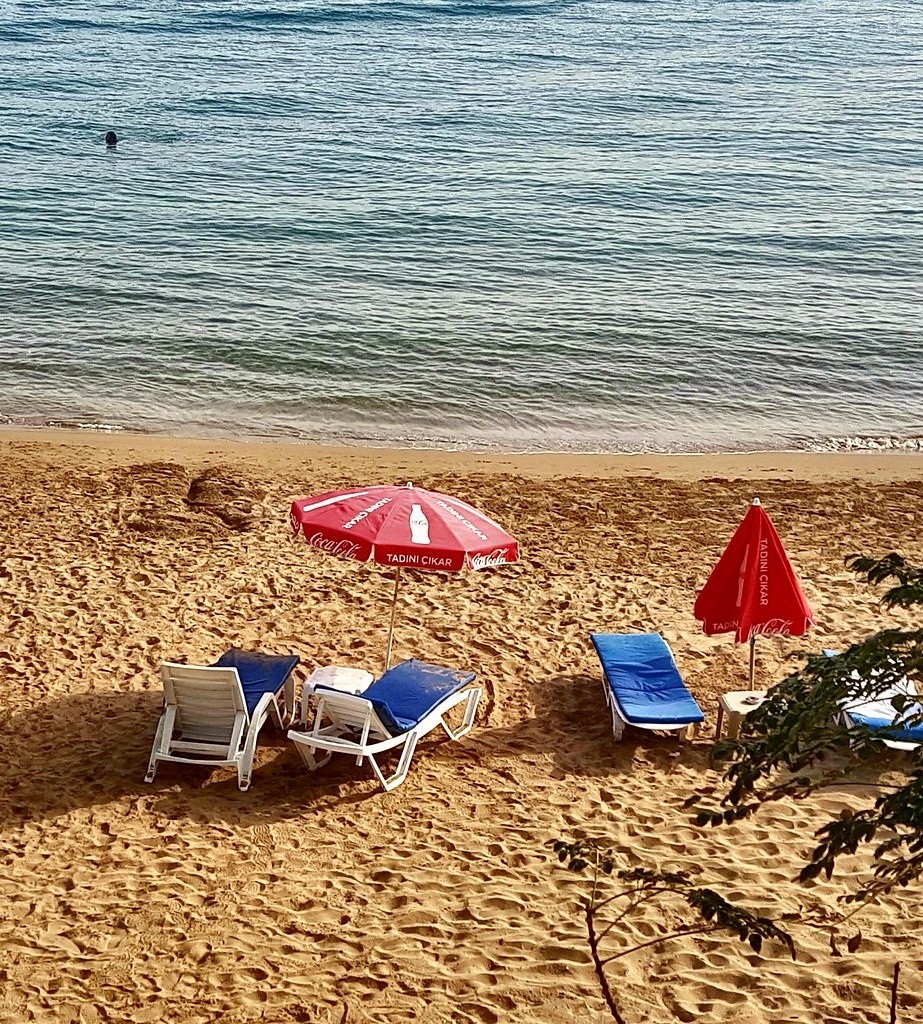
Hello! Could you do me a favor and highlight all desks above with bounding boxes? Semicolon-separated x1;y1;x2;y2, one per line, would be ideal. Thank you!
716;691;788;764
301;666;374;729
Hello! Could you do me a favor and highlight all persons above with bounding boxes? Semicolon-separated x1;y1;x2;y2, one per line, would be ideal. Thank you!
104;131;119;144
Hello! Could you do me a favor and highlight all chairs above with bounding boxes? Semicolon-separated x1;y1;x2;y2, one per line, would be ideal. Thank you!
143;647;300;791
591;633;704;743
287;658;482;792
820;650;923;759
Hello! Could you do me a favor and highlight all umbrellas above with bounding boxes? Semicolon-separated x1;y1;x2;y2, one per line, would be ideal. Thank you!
288;481;522;675
695;498;818;691
